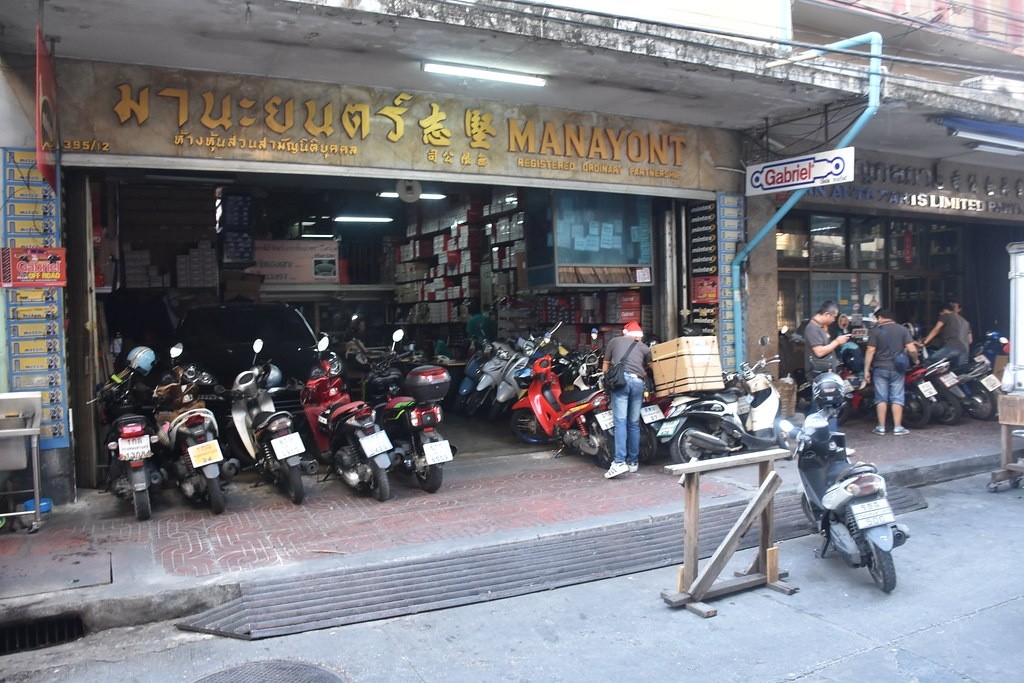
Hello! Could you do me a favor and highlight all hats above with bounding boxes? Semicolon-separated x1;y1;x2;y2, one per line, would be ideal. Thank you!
623;320;643;338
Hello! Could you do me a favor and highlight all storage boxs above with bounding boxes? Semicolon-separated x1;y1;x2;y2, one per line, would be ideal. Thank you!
651;335;724;396
404;365;450;403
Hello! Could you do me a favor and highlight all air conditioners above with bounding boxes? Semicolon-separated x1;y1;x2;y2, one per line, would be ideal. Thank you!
960;75;1024;101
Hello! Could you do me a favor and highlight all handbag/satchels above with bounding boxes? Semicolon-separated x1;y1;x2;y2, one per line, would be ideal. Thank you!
894;353;910;372
604;362;627;392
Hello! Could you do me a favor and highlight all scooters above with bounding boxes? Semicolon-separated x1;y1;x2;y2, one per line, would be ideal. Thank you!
835;328;1010;428
211;338;319;504
457;321;792;469
779;402;909;592
143;341;239;514
85;365;169;520
287;335;401;501
356;328;457;492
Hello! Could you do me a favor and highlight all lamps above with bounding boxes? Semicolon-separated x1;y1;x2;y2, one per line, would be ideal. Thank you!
145;173;236;183
333;214;394;223
945;127;1024;149
378;189;447;201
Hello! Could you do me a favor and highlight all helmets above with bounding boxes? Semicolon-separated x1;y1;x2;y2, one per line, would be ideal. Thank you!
812;372;845;408
126;346;156;376
256;360;282;389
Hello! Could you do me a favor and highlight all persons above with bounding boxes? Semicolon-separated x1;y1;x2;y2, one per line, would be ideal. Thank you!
601;320;653;479
831;313;854;355
864;307;919;436
950;301;961;314
912;303;973;398
804;300;855;456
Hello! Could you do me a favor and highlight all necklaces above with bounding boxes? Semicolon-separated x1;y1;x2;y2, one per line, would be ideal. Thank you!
811;317;824;328
881;321;896;323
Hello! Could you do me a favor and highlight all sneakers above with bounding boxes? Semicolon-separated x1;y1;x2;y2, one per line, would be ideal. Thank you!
628;460;639;473
873;427;887;436
604;460;630;479
894;426;910;435
